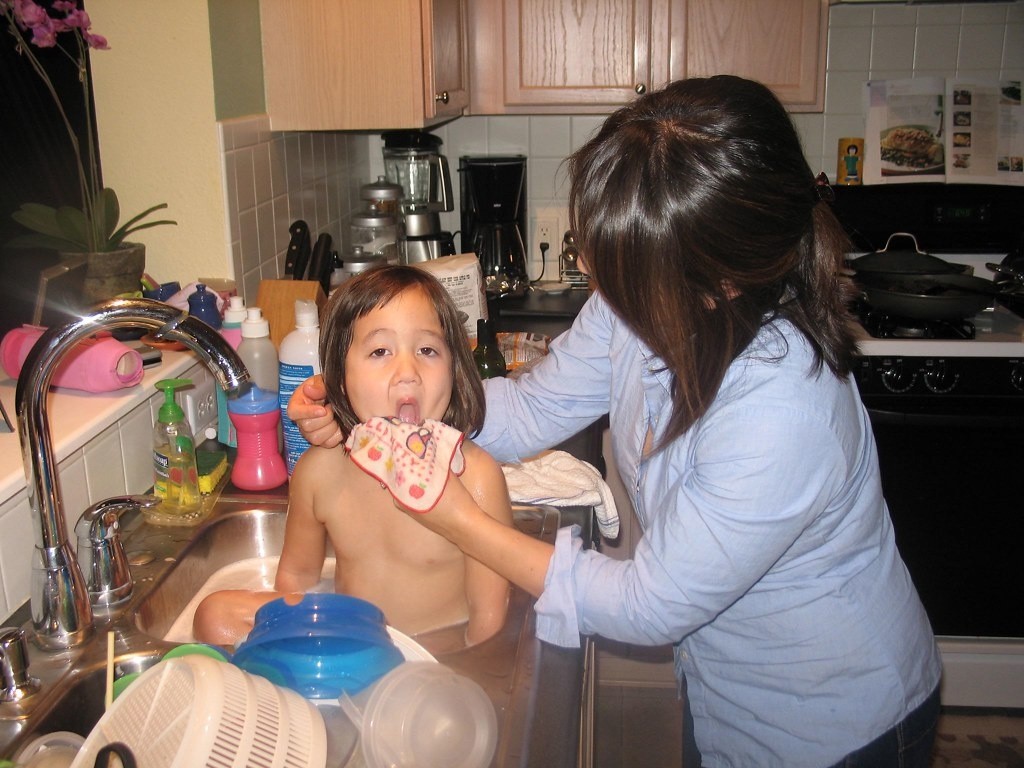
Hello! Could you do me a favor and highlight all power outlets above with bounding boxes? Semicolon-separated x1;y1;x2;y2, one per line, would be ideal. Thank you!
531;215;558;261
188;375;217;436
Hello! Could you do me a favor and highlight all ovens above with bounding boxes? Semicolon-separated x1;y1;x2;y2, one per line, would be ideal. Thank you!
854;402;1024;647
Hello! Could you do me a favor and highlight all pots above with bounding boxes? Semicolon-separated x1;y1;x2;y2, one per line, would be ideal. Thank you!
844;232;999;322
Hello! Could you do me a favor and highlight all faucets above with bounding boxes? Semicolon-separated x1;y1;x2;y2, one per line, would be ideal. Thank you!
15;295;251;647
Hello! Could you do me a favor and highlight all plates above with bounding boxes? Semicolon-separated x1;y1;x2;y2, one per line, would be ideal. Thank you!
881;125;944;174
140;332;186;350
536;284;571;294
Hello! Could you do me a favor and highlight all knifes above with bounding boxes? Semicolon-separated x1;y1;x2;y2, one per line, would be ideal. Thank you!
281;221;334;298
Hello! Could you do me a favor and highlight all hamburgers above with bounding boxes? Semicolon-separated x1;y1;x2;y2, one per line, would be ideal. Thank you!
879;127;939;167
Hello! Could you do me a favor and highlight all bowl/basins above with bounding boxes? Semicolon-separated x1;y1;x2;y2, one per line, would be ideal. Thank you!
362;657;499;767
232;594;403;698
16;731;86;768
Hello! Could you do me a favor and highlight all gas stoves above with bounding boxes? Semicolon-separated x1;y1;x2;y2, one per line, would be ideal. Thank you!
810;182;1024;420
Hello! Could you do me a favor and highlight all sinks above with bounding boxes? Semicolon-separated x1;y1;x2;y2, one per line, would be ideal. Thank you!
135;508;521;655
0;654;171;768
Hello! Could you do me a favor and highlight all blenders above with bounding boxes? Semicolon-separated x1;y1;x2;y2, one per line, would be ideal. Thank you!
378;132;456;269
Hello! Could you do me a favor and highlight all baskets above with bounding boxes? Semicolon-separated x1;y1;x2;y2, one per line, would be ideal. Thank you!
70;654;328;768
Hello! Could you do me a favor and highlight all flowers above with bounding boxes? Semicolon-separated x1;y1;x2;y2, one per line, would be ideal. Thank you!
0;0;175;243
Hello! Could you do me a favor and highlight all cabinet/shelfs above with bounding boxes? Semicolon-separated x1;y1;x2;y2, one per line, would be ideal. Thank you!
260;0;470;134
466;0;831;118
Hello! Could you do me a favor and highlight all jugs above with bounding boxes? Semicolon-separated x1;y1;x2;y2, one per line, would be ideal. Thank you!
472;223;528;290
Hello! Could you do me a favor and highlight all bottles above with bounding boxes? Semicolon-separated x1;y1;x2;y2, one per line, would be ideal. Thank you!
227;382;286;490
361;176;407;263
348;211;400;267
472;319;507;380
330;251;346;287
215;295;249;446
236;308;283;454
277;299;323;477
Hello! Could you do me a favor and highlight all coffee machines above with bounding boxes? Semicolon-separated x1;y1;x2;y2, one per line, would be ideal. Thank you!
458;155;531;299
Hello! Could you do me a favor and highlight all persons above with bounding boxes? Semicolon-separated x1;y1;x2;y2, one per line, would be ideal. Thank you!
195;266;515;655
288;74;942;767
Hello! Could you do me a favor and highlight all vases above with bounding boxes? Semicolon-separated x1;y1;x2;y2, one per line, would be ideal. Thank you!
35;239;147;335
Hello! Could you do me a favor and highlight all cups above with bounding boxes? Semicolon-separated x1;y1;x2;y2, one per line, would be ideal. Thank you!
187;282;223;333
144;282;180;302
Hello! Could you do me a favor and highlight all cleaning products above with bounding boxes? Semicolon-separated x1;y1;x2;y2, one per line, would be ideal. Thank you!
214;283;325;492
153;378;202;514
